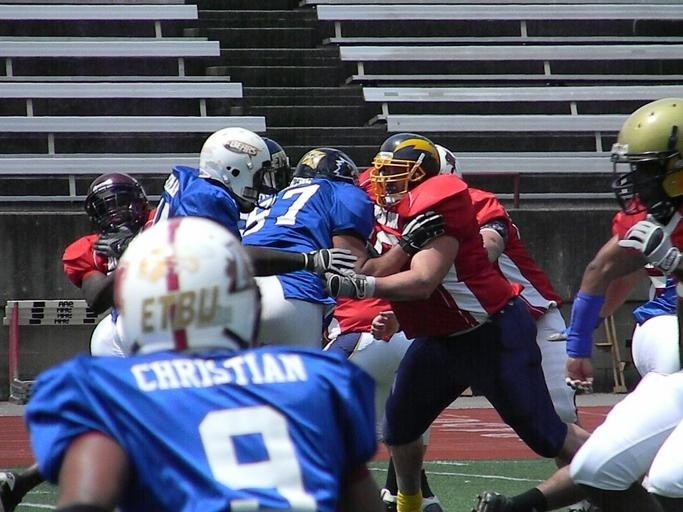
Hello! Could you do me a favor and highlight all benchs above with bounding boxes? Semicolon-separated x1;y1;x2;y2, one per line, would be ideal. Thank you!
1;79;245;116
362;86;678;133
443;150;641;210
338;46;665;85
0;149;202;215
0;110;266;156
0;1;199;40
316;1;665;44
0;33;230;84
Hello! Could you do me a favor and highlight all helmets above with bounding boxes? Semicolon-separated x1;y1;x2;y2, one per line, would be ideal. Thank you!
610;97;683;221
113;217;260;354
259;136;289;195
84;171;149;235
290;147;360;188
196;126;271;213
370;132;440;207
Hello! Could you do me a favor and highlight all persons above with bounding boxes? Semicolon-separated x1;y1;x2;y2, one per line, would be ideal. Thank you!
25;217;383;512
324;297;431;512
62;172;156;314
568;96;682;512
435;142;581;430
567;191;683;394
236;135;291;235
471;460;584;510
324;133;665;512
241;146;409;353
90;126;356;356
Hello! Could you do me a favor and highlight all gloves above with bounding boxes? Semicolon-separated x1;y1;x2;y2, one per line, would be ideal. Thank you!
398;211;446;256
617;221;681;274
322;270;375;302
94;233;129;257
564;355;595;393
302;247;357;276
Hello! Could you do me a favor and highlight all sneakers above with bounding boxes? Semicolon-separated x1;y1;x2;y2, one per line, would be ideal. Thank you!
0;471;26;512
470;491;510;512
420;495;444;512
380;488;397;512
